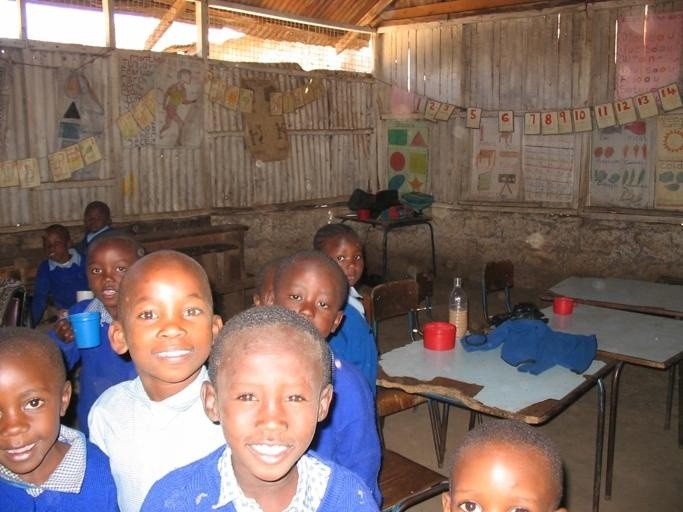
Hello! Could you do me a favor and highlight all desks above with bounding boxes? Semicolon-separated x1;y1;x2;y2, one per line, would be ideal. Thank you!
540;274;683;317
523;303;683;500
376;330;617;512
333;213;436;279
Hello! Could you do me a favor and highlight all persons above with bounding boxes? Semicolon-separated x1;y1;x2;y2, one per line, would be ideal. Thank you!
251;259;280;309
313;222;378;397
87;250;226;512
141;307;381;512
1;326;119;512
273;250;380;510
439;420;566;510
30;225;90;332
49;228;145;438
81;202;113;253
158;68;198;145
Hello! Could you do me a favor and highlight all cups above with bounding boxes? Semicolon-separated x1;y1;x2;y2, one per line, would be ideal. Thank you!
64;310;101;350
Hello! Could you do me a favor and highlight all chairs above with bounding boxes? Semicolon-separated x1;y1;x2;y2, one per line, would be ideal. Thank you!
371;280;481;468
407;272;436;338
483;260;515;325
378;447;450;512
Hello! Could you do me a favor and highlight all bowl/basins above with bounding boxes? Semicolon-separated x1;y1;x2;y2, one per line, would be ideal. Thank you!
423;321;456;350
357;209;370;219
553;297;576;315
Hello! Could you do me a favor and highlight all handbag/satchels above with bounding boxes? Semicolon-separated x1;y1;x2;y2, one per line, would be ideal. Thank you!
376;204;415;223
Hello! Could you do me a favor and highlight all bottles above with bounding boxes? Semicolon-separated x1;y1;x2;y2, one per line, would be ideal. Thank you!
449;277;469;339
